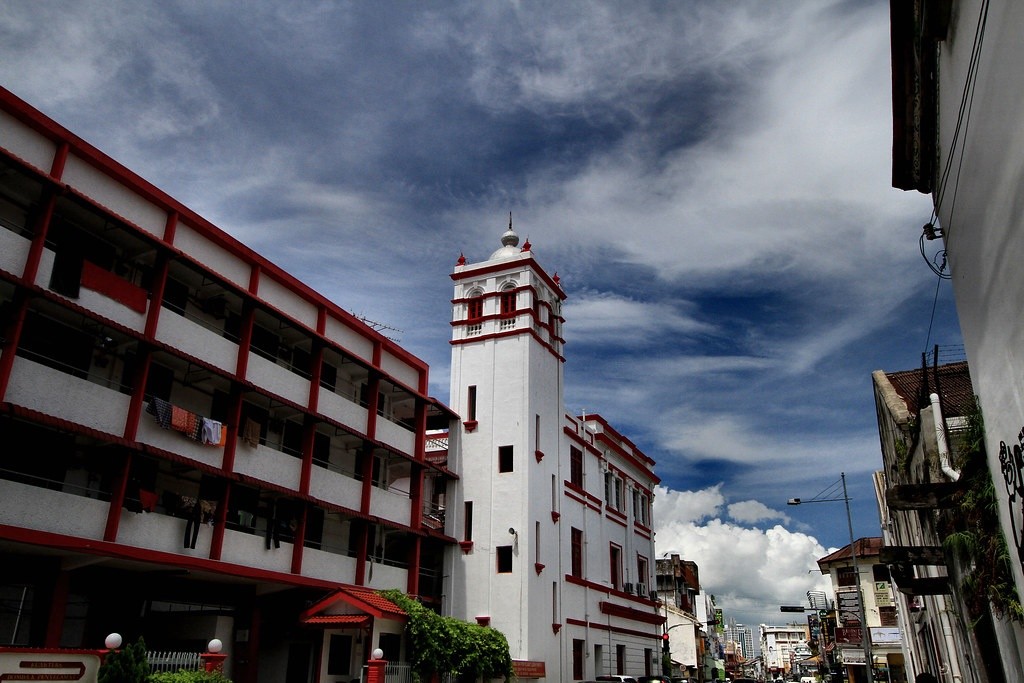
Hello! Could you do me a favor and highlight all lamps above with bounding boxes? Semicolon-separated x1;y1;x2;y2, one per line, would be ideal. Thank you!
372;648;384;660
208;639;223;653
508;527;518;544
105;633;122;649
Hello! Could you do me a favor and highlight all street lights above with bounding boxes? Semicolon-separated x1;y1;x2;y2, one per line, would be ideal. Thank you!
788;498;874;683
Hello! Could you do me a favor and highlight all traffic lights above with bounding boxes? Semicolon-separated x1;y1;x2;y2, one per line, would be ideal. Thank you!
707;620;719;626
663;634;669;653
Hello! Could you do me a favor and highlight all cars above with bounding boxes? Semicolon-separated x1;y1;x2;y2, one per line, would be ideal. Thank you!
596;674;700;683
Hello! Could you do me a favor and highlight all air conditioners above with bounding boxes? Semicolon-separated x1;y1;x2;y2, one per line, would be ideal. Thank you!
651;590;657;599
638;583;646;596
625;583;633;592
